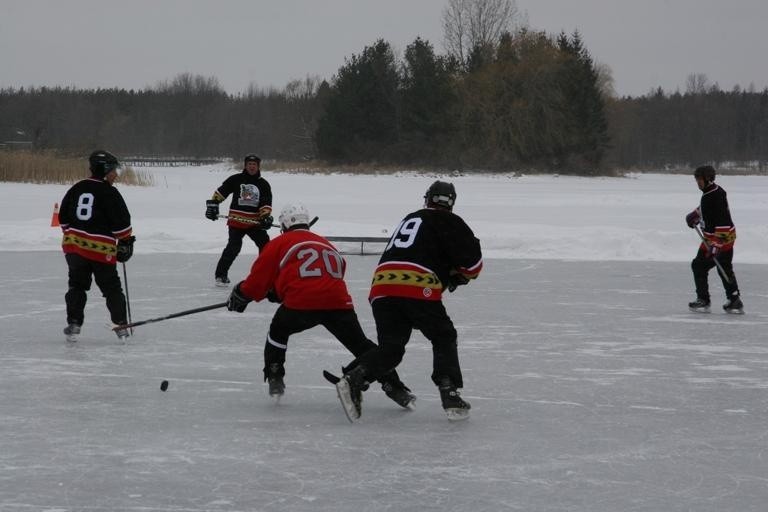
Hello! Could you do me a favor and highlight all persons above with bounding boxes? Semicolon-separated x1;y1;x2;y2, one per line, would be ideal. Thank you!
686;166;744;309
343;181;484;418
57;150;136;335
227;204;417;407
205;152;272;283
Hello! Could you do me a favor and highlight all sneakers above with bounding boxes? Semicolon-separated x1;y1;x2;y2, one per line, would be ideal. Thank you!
216;275;230;283
65;321;81;334
689;297;710;307
116;321;132;336
724;296;743;309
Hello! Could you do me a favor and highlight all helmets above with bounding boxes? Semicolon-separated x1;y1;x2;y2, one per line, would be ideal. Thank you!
278;203;309;230
694;166;715;180
89;151;119;176
245;153;261;165
426;180;456;209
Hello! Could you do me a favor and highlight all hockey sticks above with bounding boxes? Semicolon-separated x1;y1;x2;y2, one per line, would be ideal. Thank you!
122;261;136;335
322;370;370;391
692;222;735;285
112;303;228;331
218;215;318;230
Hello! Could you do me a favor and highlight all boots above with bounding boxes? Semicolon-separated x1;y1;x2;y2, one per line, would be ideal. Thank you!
265;362;285;393
436;376;471;409
382;382;415;406
343;366;368;417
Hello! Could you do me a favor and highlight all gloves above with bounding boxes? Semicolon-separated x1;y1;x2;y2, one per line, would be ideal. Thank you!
226;282;252;312
116;236;135;261
687;209;701;228
709;241;720;256
206;199;219;221
261;215;273;230
